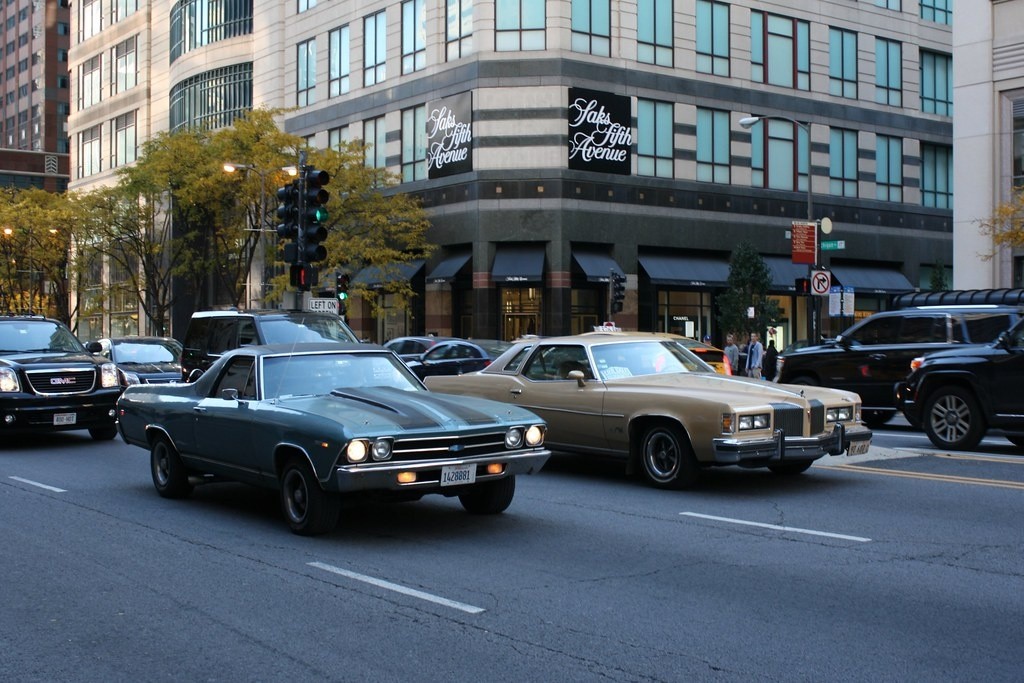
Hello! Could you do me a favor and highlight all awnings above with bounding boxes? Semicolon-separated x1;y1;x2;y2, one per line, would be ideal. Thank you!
349;259;425;288
830;266;914;294
638;254;730;287
492;245;545;283
760;257;813;291
425;253;473;283
316;265;350;291
572;251;627;282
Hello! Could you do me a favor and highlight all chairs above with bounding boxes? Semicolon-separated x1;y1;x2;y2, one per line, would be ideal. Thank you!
557;361;593;381
221;373;255;400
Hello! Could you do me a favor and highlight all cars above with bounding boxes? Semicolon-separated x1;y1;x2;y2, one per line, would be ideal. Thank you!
423;335;873;490
383;336;529;384
90;336;186;391
116;342;550;539
534;330;732;381
893;318;1024;453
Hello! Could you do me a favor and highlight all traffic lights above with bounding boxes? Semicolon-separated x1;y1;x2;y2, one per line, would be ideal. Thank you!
794;277;810;297
276;179;299;238
335;271;348;314
300;165;329;263
285;242;321;293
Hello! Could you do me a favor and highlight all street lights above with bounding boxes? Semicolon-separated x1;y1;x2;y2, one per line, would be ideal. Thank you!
739;115;813;344
223;161;296;310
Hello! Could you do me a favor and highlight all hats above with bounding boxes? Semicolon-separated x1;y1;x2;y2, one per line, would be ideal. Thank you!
703;335;712;340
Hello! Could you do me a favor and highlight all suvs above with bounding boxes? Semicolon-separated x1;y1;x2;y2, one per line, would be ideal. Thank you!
776;303;1024;433
182;309;391;401
0;315;122;442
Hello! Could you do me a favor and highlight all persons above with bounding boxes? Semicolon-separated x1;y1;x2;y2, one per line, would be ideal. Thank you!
765;340;778;380
745;331;763;379
724;333;738;376
704;335;711;346
